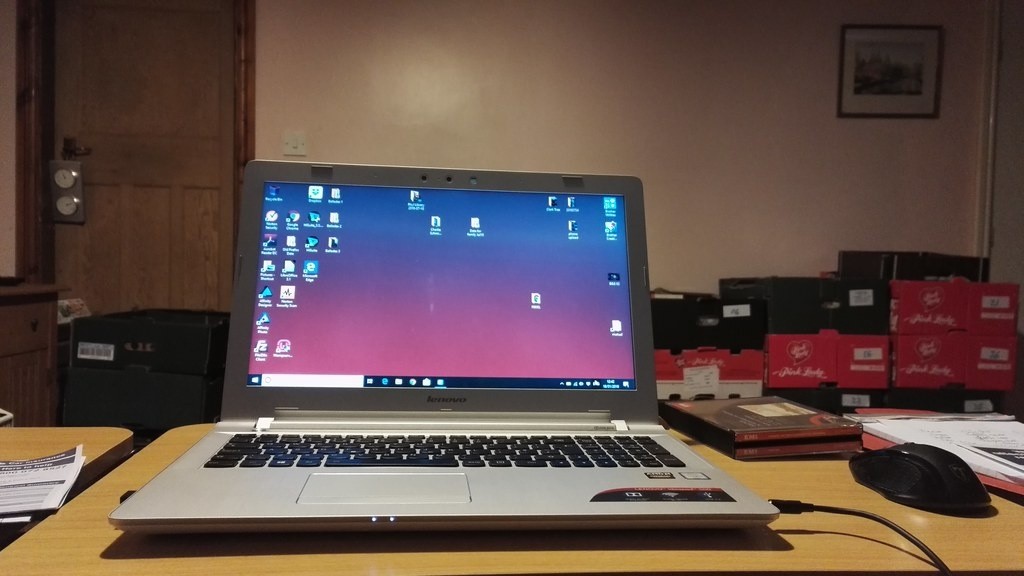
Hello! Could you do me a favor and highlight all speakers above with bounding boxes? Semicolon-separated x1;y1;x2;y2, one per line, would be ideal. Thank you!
56;364;212;450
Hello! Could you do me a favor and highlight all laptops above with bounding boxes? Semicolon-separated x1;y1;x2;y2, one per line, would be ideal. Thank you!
106;157;779;534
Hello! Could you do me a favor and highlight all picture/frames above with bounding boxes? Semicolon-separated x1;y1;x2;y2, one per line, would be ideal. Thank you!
836;22;944;120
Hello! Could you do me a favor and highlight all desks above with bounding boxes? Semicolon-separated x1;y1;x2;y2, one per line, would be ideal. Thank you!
0;425;134;552
0;422;1024;576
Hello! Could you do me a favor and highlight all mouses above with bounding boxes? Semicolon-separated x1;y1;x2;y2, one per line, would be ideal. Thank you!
847;441;993;515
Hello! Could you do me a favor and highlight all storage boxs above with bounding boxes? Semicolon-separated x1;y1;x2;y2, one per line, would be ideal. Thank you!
649;252;1019;413
61;366;221;433
70;308;231;375
